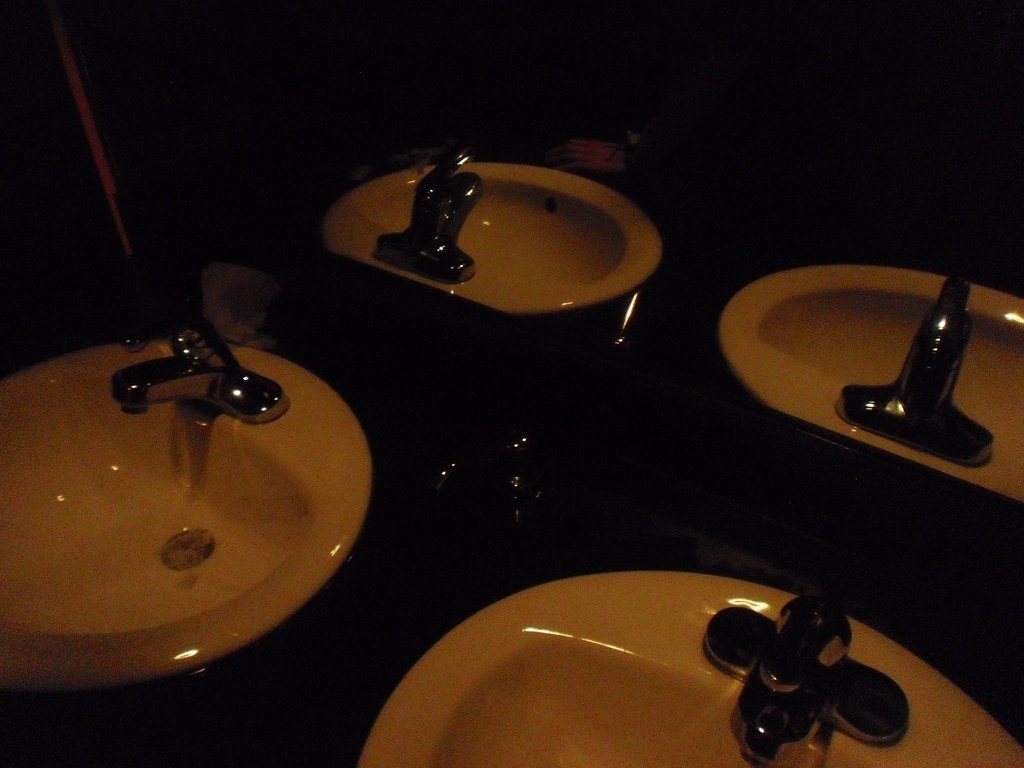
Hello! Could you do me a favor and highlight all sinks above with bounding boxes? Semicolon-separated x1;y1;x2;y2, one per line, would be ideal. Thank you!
360;570;1024;768
1;337;370;690
720;264;1024;504
320;161;662;314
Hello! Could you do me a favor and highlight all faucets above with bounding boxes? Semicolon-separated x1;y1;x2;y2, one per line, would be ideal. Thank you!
378;143;485;280
701;599;909;765
110;321;287;421
837;279;992;465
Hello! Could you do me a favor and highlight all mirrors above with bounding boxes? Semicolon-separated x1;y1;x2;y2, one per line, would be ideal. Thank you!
54;0;1024;511
